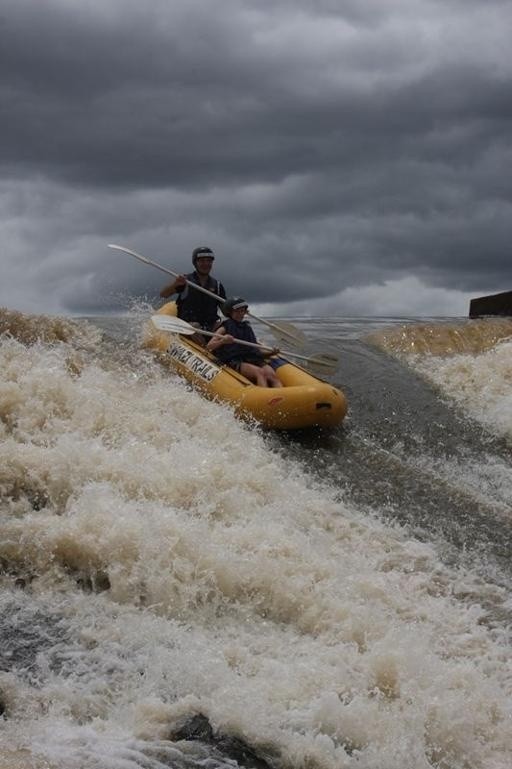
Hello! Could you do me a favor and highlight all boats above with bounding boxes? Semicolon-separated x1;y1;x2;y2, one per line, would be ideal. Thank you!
144;295;350;433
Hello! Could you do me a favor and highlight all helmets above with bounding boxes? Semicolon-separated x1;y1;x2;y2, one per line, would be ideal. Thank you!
222;296;248;317
192;247;214;264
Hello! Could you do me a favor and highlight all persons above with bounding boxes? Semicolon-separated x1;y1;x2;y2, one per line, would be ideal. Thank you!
161;247;250;346
207;296;285;388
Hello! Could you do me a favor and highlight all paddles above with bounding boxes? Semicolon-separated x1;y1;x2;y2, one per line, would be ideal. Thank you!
109;244;309;350
151;316;339;374
